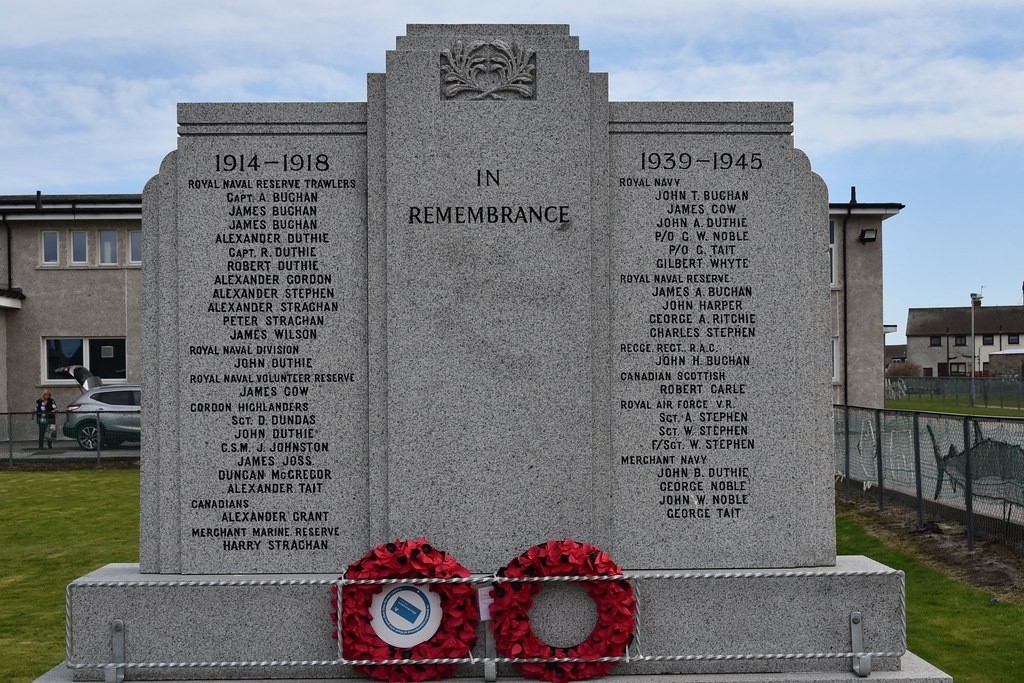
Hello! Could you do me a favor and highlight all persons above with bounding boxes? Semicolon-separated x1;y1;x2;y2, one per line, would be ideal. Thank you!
31;390;58;452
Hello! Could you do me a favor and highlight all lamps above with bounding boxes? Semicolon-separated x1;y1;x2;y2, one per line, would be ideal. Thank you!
857;227;878;245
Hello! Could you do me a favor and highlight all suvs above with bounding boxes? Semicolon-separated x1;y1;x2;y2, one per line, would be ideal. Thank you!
55;365;140;452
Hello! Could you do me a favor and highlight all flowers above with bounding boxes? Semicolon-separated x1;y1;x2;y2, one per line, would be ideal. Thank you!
490;540;640;683
327;539;479;683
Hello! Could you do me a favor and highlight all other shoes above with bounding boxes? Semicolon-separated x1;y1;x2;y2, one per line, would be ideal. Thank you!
38;447;43;451
48;443;53;450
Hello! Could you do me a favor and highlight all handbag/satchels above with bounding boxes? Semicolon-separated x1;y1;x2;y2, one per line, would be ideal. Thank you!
44;424;56;440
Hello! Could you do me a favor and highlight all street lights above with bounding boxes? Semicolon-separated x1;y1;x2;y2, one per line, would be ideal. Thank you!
970;292;984;407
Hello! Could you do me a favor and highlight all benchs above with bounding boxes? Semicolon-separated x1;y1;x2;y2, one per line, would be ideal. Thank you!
906;387;934;401
